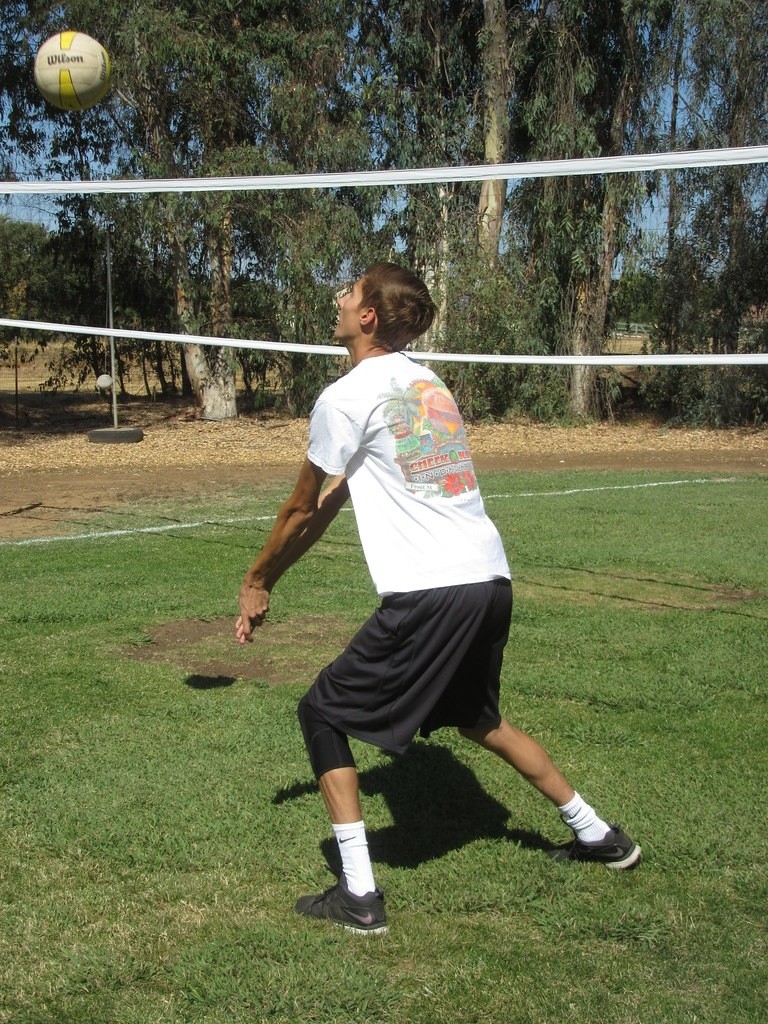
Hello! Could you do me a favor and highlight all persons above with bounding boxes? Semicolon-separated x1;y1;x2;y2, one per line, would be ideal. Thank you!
234;264;642;934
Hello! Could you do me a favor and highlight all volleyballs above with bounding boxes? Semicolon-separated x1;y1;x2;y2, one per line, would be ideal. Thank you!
32;29;114;114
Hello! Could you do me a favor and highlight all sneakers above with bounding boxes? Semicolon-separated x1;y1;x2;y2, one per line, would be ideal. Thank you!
543;814;641;868
295;870;389;937
